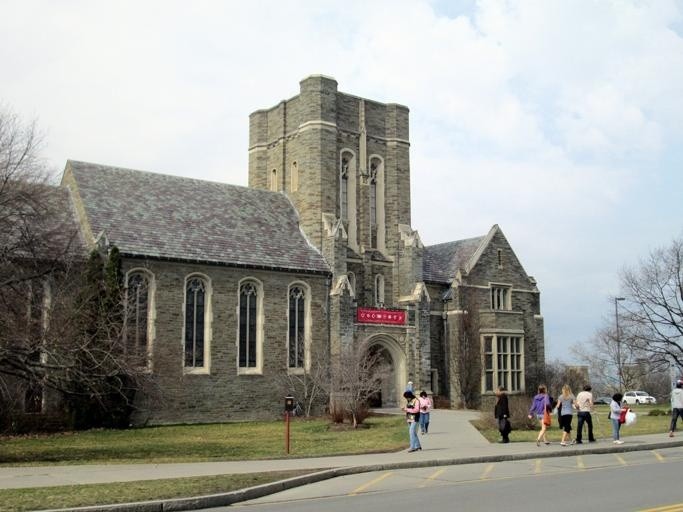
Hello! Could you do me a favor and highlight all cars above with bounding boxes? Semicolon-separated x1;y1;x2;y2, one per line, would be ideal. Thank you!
593;397;612;405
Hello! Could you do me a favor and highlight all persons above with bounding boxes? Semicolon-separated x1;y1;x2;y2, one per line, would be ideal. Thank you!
667;383;683;437
527;383;552;446
401;390;421;453
556;383;579;445
493;386;510;443
574;384;595;443
609;392;626;444
417;391;431;434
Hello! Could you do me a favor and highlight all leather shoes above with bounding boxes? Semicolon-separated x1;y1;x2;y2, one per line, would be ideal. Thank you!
498;439;510;444
535;439;551;447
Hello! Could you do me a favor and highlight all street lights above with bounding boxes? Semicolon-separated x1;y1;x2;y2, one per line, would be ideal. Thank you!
615;297;626;384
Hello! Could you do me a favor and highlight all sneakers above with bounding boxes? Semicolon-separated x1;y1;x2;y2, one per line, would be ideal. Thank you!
560;439;596;446
613;440;625;445
408;447;422;453
668;431;675;437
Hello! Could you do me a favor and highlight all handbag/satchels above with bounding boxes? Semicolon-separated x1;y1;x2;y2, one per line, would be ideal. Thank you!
498;416;511;435
543;410;552;427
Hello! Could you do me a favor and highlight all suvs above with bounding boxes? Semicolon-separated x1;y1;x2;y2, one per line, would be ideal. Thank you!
621;390;656;405
